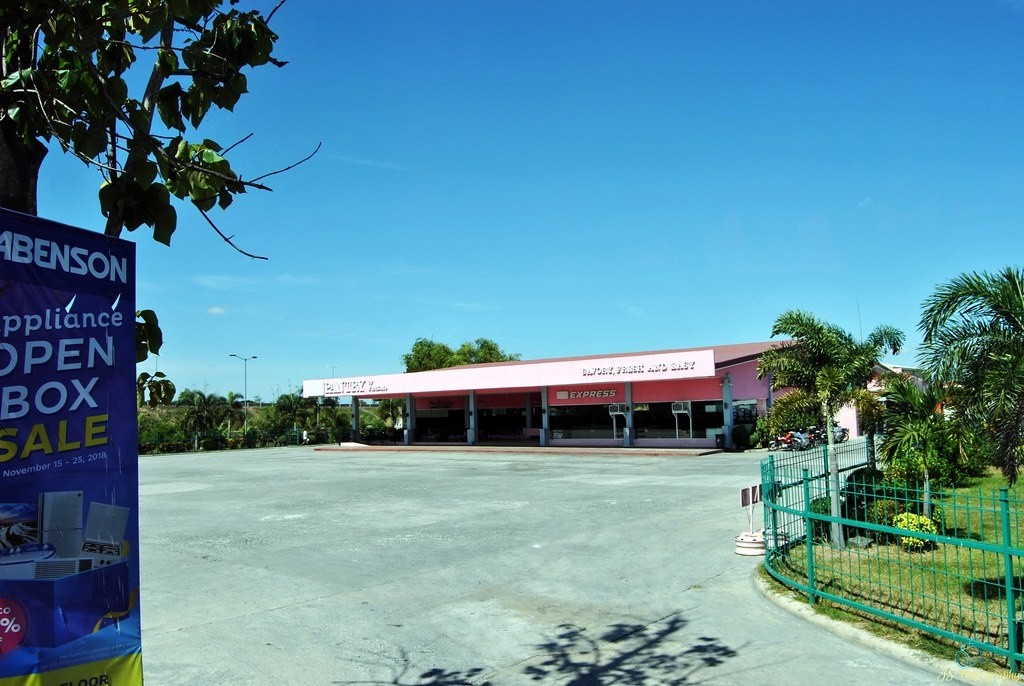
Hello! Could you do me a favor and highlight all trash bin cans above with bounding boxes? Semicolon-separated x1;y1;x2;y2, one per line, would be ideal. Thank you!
715;434;726;449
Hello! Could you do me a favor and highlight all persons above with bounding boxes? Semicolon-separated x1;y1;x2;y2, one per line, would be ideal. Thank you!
301;429;310;447
315;428;329;443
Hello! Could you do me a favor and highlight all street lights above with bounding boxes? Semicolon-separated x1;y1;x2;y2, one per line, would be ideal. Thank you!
229;352;259;435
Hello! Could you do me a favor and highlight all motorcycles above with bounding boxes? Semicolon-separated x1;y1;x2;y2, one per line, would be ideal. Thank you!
766;420;851;452
